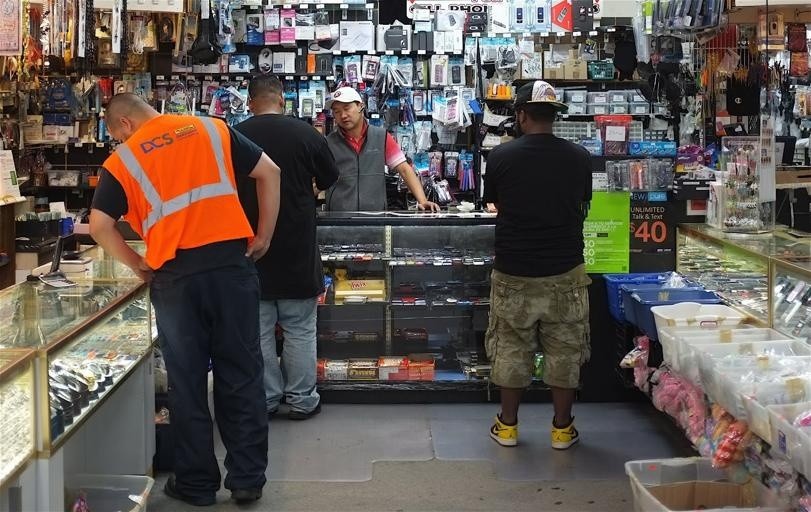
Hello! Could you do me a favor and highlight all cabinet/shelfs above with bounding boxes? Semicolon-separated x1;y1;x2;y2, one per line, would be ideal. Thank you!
676;223;810;348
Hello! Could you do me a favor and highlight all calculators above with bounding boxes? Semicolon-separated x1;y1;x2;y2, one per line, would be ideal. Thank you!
39;272;79;288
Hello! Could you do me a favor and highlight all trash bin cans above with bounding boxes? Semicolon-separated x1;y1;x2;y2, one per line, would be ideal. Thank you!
63;472;156;511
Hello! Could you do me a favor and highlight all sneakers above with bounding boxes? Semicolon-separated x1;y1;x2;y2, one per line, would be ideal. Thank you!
550;415;580;449
164;475;216;505
489;413;519;446
228;482;261;500
288;402;322;421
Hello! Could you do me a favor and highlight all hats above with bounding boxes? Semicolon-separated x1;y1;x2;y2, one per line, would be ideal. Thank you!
514;81;568;112
325;88;361;111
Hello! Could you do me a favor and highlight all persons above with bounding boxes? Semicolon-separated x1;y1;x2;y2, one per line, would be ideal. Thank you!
483;81;592;449
88;92;282;507
312;87;441;242
230;73;341;420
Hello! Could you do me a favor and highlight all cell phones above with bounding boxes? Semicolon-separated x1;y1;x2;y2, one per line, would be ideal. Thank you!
677;243;768;316
319;243;495;266
392;279;491;306
155;5;526;177
